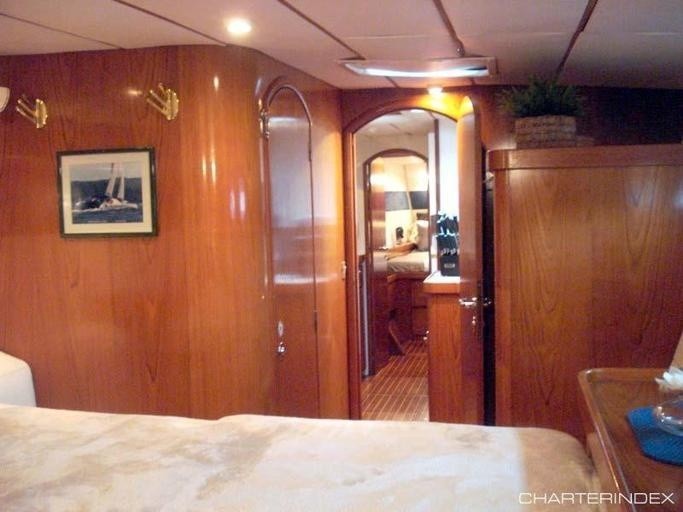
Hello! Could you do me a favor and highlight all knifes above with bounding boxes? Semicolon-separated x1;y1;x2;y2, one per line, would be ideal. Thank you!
437;216;458;257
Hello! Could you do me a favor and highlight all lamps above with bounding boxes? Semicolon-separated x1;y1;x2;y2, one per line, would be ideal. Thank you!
339;56;490;78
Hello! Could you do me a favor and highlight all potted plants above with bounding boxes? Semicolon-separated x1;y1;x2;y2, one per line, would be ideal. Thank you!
496;75;587;149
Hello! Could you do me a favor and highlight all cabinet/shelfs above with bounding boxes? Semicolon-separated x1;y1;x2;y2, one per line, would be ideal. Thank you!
377;277;428;369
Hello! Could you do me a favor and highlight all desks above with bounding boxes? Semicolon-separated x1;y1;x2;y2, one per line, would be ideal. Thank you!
577;364;682;511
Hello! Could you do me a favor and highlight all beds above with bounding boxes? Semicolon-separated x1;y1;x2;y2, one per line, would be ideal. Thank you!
0;349;611;512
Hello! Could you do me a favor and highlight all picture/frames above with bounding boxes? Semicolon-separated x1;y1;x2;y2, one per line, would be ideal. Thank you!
57;147;159;238
